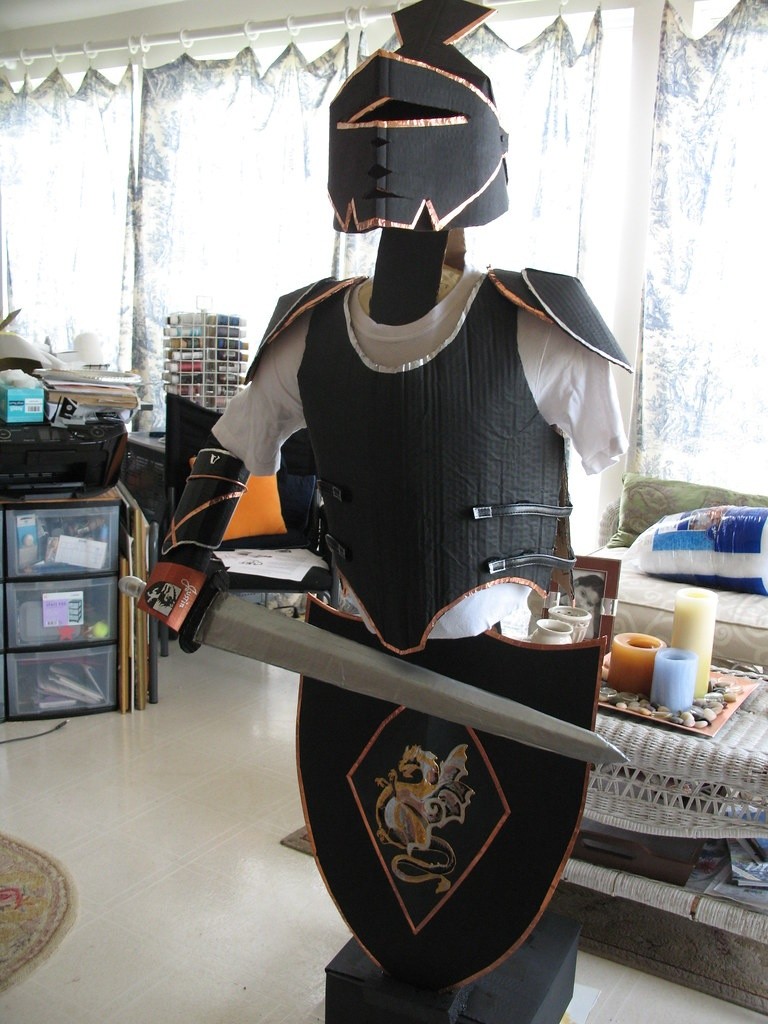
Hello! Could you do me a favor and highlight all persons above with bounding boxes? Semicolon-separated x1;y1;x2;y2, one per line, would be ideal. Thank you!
137;1;632;998
572;575;603;611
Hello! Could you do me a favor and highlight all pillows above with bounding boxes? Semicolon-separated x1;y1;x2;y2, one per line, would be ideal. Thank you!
608;472;768;548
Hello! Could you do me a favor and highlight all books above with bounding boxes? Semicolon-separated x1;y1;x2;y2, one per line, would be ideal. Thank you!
32;368;141;422
722;807;768;889
27;658;106;709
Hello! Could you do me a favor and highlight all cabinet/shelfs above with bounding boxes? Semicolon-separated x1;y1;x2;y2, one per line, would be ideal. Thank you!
0;484;148;723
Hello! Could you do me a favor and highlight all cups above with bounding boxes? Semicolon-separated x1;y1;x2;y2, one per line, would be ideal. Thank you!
548;605;591;642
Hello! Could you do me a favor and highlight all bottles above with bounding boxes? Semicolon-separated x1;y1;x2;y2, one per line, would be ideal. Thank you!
531;619;573;644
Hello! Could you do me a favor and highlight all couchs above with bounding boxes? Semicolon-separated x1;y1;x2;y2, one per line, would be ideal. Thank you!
584;497;768;674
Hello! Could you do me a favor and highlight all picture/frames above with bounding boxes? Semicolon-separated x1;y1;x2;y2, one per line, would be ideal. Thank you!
573;555;621;652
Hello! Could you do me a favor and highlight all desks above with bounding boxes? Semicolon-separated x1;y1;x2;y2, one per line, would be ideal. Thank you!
562;665;768;942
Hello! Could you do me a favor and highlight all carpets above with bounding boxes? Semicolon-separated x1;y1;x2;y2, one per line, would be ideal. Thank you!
0;833;78;995
280;822;768;1014
